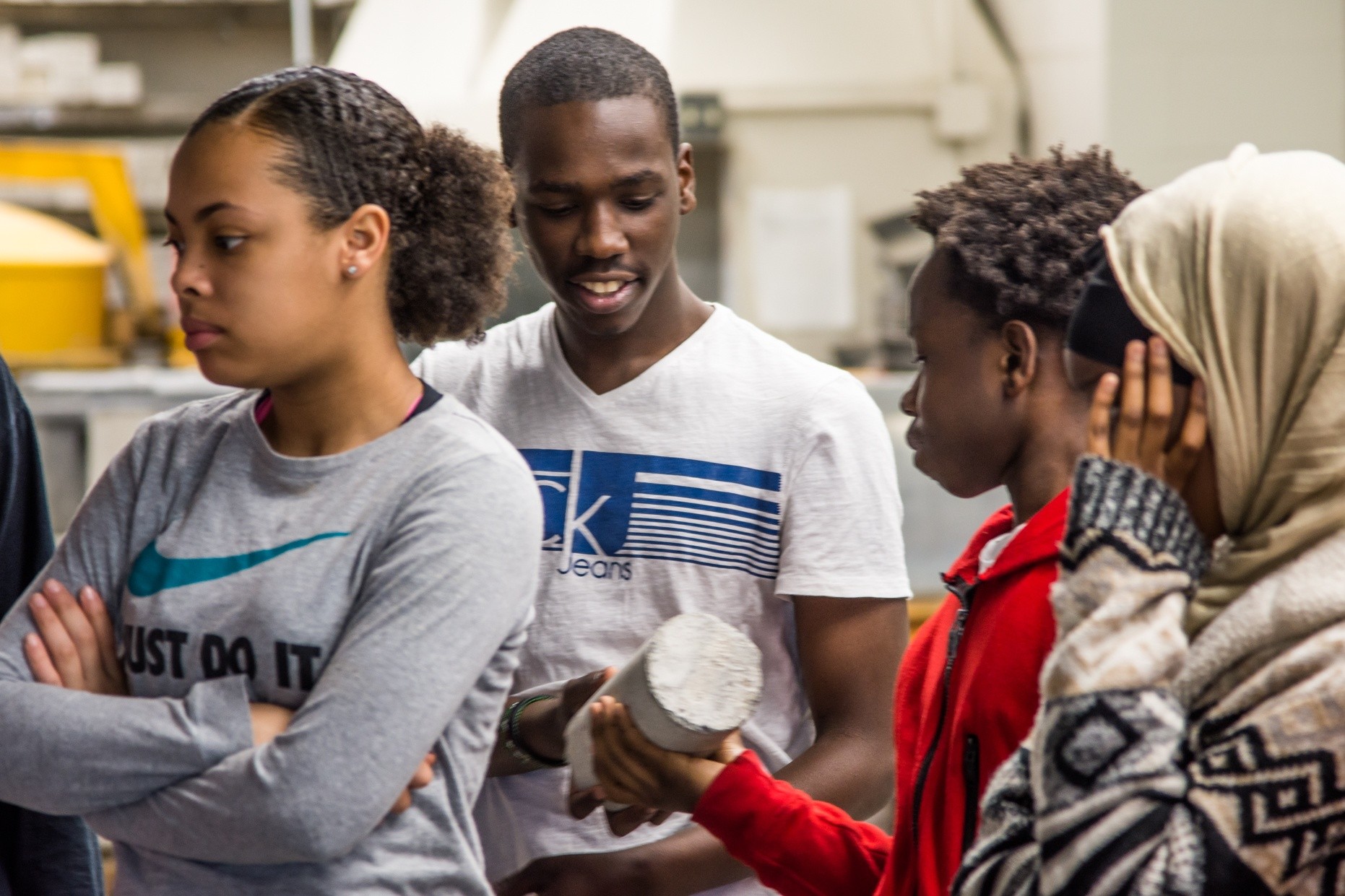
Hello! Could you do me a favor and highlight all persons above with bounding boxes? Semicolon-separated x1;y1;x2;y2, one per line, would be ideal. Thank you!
587;146;1148;896
953;142;1345;896
0;356;101;896
402;28;914;896
0;69;546;896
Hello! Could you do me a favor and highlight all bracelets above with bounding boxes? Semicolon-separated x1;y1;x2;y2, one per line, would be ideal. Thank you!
499;693;568;772
